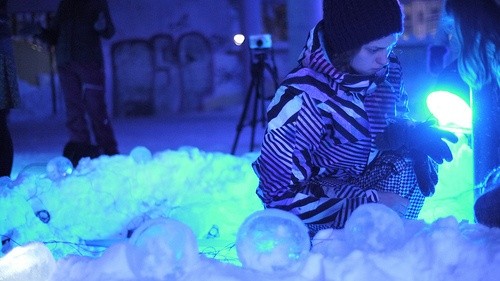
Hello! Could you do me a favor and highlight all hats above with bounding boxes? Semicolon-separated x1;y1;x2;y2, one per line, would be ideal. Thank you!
322;0;403;57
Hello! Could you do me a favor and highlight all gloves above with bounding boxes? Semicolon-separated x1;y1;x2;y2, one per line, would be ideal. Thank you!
376;119;458;197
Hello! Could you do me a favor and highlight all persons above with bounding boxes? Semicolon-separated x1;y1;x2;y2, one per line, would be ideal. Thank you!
0;0;23;177
252;0;500;239
32;0;120;161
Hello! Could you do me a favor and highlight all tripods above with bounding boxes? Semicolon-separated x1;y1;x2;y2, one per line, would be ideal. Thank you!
230;52;280;155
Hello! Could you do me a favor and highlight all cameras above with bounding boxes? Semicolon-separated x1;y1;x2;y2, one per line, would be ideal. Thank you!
249;34;271;50
